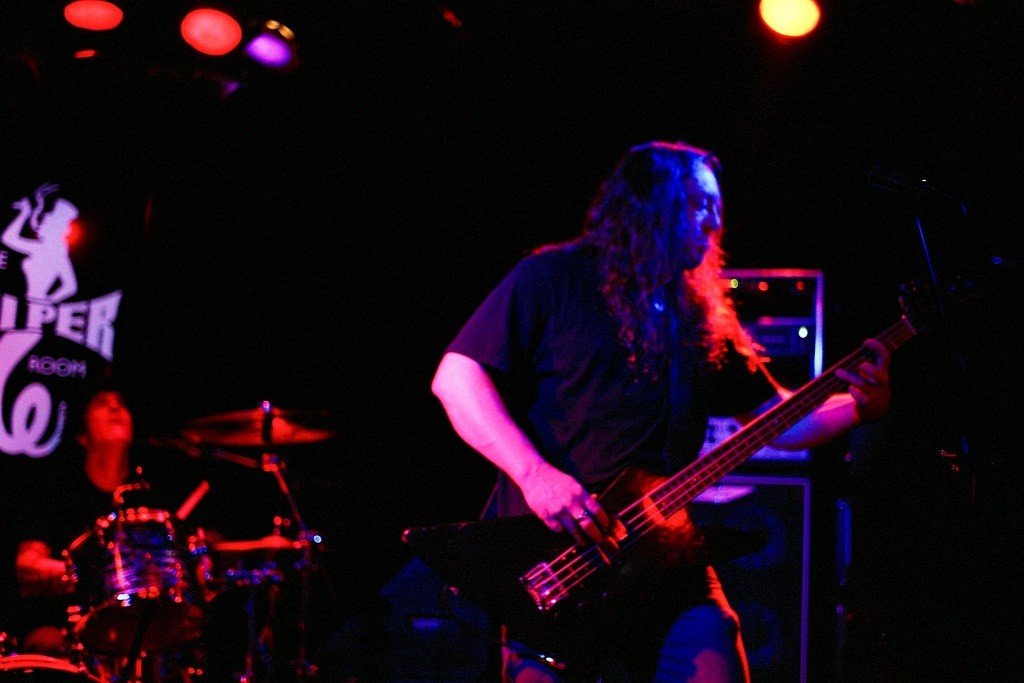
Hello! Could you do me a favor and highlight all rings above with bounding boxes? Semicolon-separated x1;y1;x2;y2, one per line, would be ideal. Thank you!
573;512;586;526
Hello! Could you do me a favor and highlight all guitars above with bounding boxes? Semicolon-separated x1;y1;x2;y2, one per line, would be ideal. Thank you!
476;252;1007;680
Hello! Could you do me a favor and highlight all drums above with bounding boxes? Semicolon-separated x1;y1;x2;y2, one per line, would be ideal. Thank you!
61;514;209;657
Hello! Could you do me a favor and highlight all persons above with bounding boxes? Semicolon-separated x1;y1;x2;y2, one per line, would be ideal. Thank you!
431;134;897;683
10;383;237;683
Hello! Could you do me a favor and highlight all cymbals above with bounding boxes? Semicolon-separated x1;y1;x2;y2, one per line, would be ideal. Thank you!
213;536;301;552
182;409;342;445
